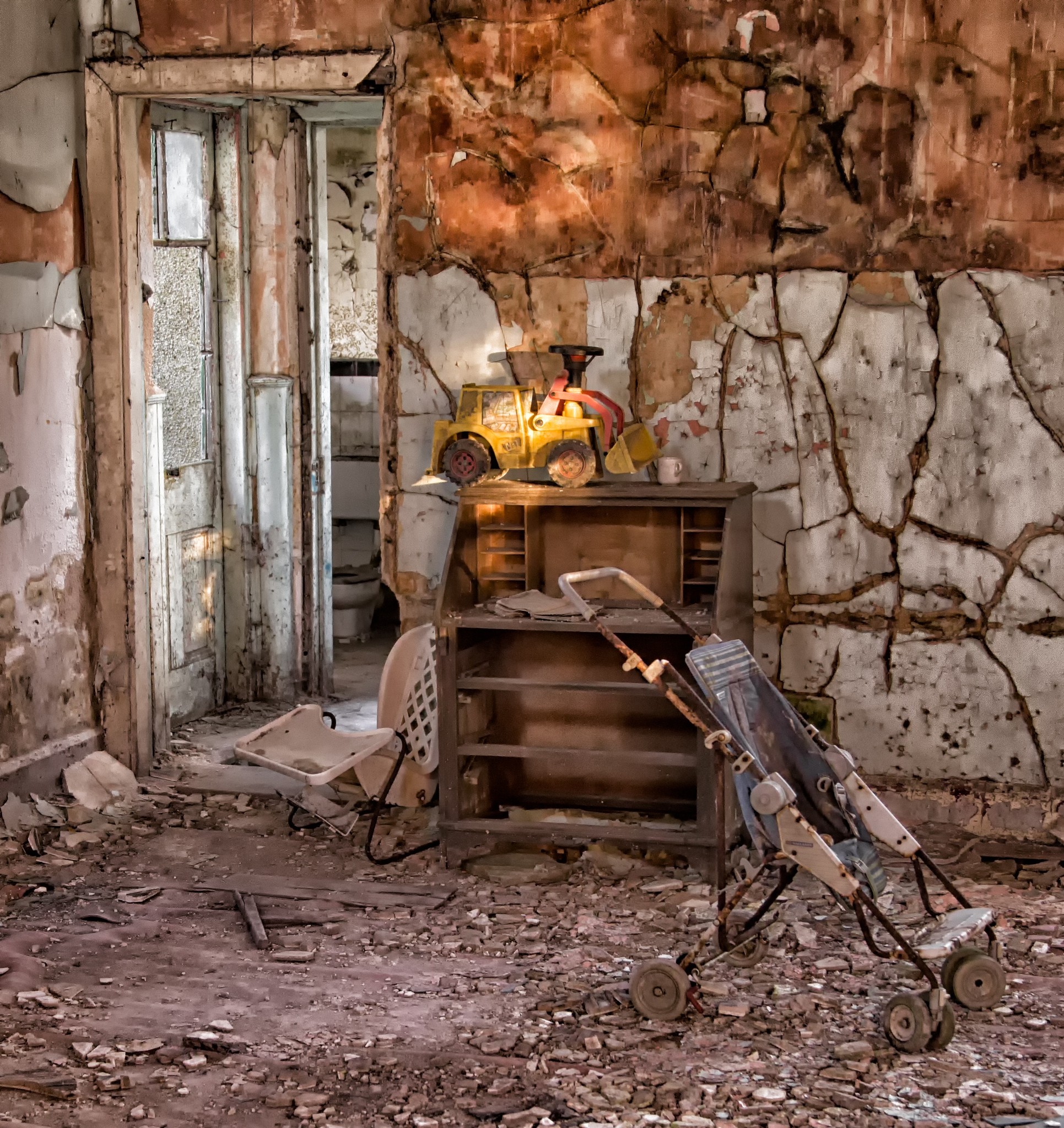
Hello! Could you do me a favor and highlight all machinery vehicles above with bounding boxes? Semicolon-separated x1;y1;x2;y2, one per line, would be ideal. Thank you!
423;338;664;490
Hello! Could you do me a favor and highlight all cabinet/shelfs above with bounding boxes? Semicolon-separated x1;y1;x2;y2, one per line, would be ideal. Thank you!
435;477;766;872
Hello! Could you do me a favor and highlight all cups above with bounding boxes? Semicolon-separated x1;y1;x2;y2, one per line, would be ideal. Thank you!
657;456;683;485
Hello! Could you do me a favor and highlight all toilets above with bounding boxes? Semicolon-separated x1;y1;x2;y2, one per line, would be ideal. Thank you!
332;561;380;645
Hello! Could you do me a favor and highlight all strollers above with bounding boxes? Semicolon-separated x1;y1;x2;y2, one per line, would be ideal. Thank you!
556;560;1009;1053
